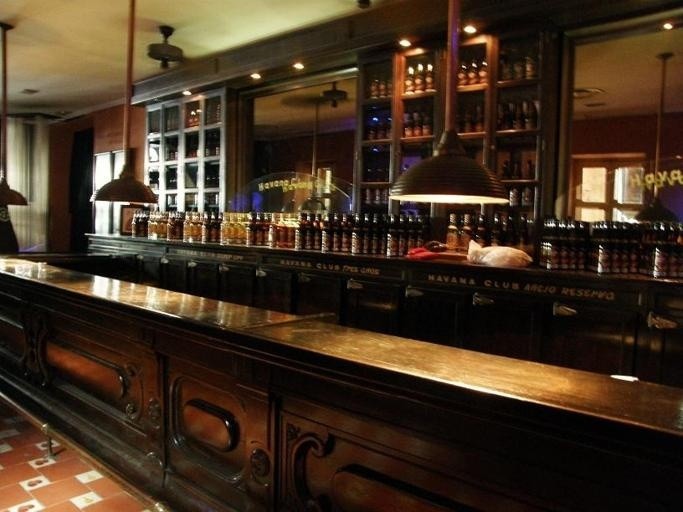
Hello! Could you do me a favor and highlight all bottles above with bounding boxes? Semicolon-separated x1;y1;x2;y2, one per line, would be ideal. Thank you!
132;208;431;255
361;49;535;258
148;101;220;210
540;215;683;279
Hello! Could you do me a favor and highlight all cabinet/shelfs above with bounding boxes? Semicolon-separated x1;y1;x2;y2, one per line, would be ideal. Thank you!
143;86;237;222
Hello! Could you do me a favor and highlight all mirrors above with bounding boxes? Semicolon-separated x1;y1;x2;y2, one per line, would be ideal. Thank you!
549;6;683;223
233;65;364;219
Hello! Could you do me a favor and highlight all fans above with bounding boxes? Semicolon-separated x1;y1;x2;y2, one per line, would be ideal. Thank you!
281;81;350;108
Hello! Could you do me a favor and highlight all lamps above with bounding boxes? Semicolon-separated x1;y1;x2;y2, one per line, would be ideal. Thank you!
635;51;679;222
90;0;159;204
0;21;29;207
388;0;510;206
280;185;299;212
298;96;327;210
147;25;183;68
